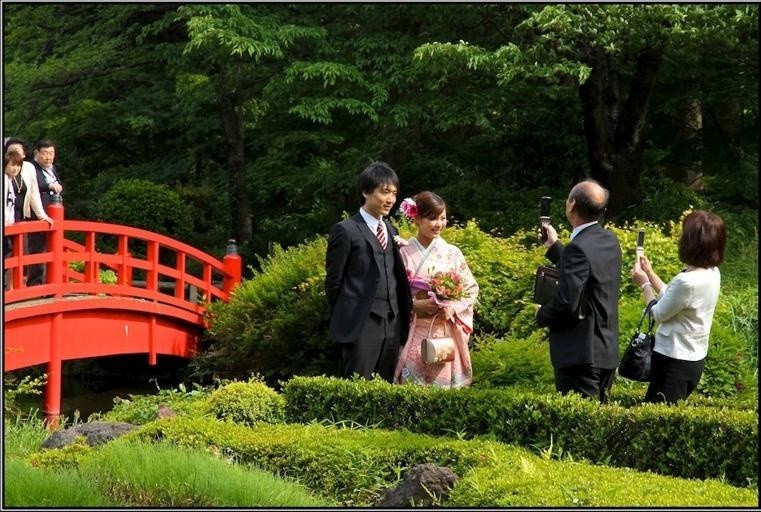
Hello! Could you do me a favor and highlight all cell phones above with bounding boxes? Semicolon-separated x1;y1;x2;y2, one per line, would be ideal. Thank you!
540;197;552;242
636;228;645;267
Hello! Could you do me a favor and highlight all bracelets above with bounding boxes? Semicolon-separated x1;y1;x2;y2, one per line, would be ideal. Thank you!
640;281;651;291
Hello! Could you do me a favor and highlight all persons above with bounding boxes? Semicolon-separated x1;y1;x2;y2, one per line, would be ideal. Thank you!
2;148;26;292
2;138;53;288
531;178;624;405
323;158;414;385
627;207;728;409
27;136;64;300
392;189;482;390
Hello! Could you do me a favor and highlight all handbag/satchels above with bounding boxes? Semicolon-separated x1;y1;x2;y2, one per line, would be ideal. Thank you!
421;336;456;366
533;266;586;321
618;331;654;383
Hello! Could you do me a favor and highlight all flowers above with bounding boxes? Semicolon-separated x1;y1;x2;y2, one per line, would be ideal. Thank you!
430;271;470;316
393;235;409;248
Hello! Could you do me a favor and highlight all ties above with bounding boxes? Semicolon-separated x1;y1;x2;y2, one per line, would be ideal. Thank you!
377;222;387;248
